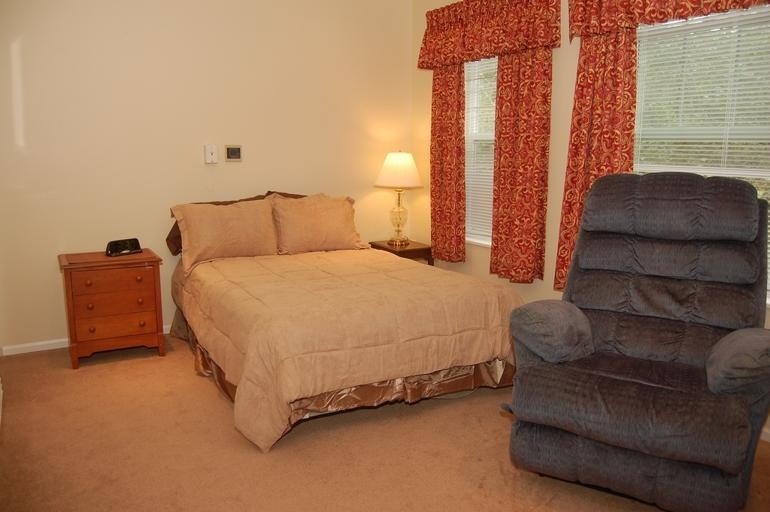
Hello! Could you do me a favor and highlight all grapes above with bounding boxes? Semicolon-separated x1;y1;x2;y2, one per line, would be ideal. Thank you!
57;249;166;369
369;238;434;265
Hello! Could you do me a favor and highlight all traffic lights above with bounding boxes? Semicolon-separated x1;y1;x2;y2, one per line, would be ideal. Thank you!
266;193;362;255
169;199;276;268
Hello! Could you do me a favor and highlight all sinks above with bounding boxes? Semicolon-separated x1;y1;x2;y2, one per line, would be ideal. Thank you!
501;172;770;512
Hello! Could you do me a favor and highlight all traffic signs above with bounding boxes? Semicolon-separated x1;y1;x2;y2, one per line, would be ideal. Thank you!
205;144;218;165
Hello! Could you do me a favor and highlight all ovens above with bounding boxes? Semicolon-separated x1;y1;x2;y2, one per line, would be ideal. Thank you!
166;190;529;455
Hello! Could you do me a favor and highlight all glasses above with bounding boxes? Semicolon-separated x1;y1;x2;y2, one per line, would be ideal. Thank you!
373;151;423;247
